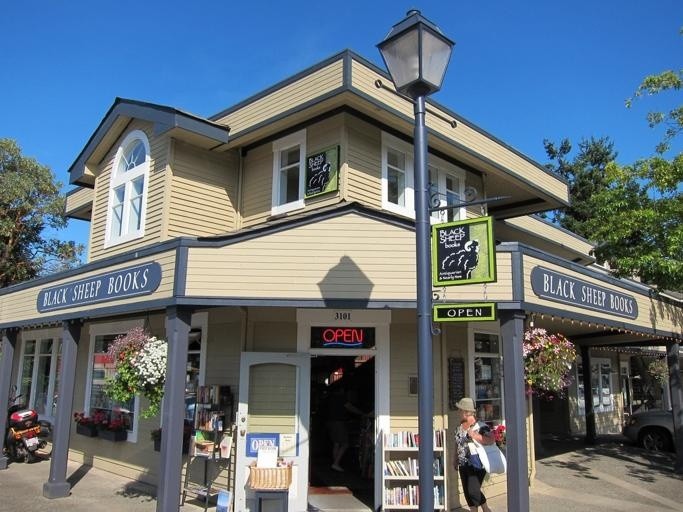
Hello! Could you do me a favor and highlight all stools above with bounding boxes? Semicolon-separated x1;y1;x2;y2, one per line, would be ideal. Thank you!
252;490;287;512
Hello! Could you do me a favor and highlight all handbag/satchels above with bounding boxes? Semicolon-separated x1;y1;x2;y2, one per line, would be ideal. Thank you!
256;441;278;468
468;443;508;474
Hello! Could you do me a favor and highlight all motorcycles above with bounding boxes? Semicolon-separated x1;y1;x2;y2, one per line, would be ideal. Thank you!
3;383;46;465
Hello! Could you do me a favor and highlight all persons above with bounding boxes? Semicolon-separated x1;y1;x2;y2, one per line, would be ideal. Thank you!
317;382;353;472
450;396;497;512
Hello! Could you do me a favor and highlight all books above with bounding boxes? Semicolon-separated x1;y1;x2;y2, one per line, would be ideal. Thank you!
256;445;278;468
214;490;232;512
381;428;445;507
188;382;231;459
182;486;221;497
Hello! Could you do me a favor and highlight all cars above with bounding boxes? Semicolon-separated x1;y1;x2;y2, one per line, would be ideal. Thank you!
624;406;674;454
87;406;129;428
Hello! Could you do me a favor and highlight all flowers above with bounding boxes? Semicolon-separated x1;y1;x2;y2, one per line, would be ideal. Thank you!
521;326;576;404
73;410;130;429
647;356;671;388
103;328;168;421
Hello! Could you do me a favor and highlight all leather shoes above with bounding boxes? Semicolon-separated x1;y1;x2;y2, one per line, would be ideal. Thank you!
332;464;344;472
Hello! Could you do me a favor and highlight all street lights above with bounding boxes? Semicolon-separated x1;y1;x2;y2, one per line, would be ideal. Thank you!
376;6;455;512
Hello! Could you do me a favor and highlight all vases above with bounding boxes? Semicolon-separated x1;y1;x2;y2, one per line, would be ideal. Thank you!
76;424;128;442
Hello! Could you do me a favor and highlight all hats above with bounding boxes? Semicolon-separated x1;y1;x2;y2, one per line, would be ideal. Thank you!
456;398;476;412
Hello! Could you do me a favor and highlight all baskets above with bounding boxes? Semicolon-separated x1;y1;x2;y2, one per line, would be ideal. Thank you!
249;461;292;490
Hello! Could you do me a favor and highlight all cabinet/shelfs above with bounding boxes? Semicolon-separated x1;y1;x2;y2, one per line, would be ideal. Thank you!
466;329;505;449
379;427;448;512
181;383;238;512
